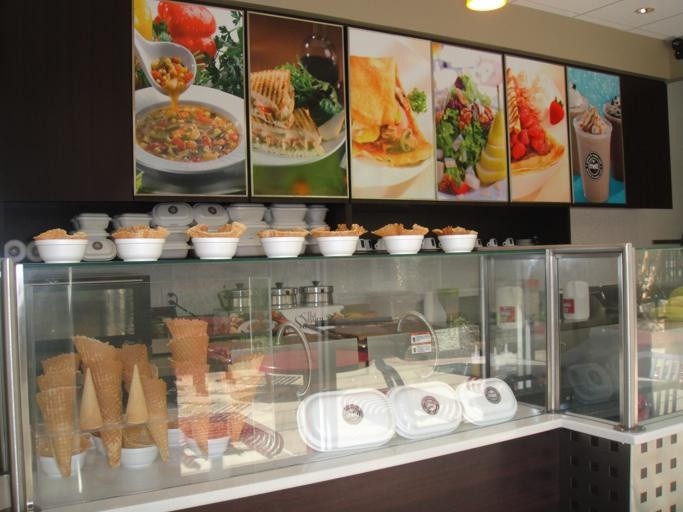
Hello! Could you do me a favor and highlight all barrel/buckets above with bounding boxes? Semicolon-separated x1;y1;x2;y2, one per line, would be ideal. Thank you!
562;278;591;322
495;284;524;331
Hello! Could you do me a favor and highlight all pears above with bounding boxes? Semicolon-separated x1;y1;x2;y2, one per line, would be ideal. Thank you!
476;84;507;185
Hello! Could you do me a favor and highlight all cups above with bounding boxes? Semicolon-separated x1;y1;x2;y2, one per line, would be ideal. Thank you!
356;238;371;249
570;96;589;176
573;115;612;204
476;237;514;247
374;239;384;251
601;101;623;183
422;238;437;248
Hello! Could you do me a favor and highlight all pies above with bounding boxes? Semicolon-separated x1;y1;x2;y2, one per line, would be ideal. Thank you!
348;55;434;166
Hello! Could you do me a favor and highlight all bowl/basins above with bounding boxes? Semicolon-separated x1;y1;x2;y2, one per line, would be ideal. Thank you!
318;235;360;256
160;241;194;260
438;234;477;254
34;238;89;264
383;234;425;255
82;237;117;261
114;238;165;262
192;237;240;260
235;238;264;257
516;239;531;245
37;426;229;480
261;236;305;259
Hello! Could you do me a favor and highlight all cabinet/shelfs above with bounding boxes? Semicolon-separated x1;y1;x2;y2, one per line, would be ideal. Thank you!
0;243;681;512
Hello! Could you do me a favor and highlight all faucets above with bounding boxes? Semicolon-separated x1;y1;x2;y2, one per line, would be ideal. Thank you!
275;320;312;397
397;310;440;378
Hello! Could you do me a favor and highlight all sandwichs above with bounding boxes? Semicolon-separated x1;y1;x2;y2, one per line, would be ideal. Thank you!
251;108;325;158
250;69;295;129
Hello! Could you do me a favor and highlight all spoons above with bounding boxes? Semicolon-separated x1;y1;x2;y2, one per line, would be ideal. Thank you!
133;31;198;96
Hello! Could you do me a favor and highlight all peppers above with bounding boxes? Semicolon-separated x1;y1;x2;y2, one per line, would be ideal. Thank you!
154;1;217;71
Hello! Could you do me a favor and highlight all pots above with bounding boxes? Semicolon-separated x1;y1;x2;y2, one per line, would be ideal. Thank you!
262;282;297;310
298;280;334;306
223;281;254;312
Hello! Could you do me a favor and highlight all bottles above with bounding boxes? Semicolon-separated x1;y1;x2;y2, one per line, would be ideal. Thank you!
470;345;513;375
518;280;539;325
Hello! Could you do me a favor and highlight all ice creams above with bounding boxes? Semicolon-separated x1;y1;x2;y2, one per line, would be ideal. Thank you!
568;81;625;203
507;67;565;176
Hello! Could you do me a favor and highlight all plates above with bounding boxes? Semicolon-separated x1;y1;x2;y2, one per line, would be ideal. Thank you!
251;103;345;167
236;318;276;334
648;304;682;321
133;84;247;176
354;113;436;189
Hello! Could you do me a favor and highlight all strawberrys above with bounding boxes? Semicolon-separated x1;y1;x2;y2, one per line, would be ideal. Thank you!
549;100;565;125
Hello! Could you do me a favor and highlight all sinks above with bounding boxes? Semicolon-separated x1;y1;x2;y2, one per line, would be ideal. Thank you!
231;385;304;401
